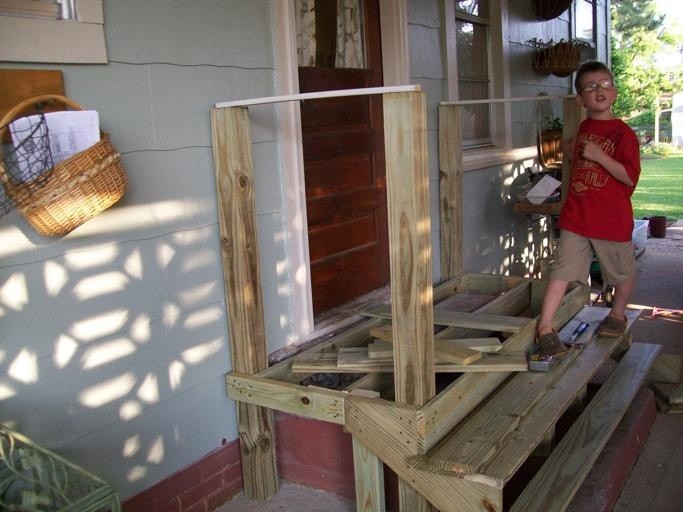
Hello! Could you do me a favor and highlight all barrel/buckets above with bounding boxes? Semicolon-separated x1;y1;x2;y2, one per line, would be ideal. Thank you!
648;216;667;239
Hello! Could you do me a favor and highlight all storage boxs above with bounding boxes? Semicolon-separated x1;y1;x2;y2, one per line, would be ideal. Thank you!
631;219;650;259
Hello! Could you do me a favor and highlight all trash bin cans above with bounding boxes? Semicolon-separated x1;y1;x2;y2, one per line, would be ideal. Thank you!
650;217;666;238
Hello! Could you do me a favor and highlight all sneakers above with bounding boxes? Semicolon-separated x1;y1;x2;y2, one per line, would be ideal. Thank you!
535;326;567;357
598;315;627;337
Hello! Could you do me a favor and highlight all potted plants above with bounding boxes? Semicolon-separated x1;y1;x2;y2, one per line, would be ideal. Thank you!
545;114;563;133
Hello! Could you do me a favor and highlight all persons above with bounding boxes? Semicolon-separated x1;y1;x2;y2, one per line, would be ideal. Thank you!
536;62;642;358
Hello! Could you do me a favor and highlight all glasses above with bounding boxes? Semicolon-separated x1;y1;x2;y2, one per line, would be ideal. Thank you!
581;79;612;92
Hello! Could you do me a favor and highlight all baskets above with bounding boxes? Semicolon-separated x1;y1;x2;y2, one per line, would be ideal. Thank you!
528;38;590;72
0;94;128;238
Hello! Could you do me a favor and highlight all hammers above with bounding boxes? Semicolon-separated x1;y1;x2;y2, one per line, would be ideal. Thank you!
563;320;589;349
